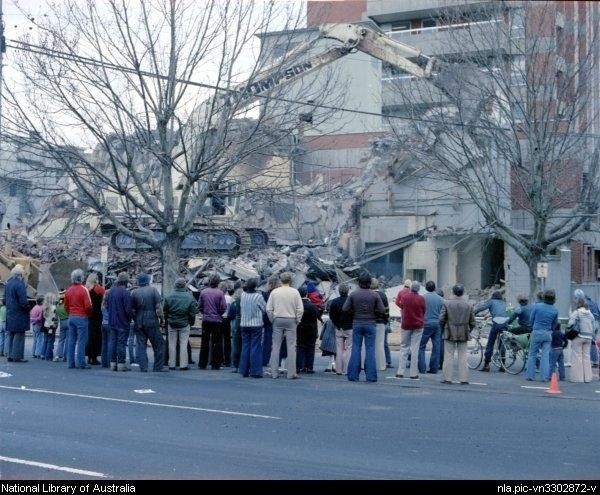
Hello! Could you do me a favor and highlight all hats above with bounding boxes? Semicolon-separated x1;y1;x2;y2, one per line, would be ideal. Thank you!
174;278;186;289
307;272;420;296
136;273;151;284
117;272;128;282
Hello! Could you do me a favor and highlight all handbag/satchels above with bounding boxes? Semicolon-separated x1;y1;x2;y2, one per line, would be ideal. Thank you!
565;322;580;340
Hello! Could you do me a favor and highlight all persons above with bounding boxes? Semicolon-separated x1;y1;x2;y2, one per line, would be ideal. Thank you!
0;263;600;385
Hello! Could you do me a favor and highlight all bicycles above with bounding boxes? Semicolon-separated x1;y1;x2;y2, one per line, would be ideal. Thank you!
467;315;525;375
491;325;541;376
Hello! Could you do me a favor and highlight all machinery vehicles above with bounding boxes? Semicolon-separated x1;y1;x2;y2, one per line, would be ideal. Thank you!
0;223;89;306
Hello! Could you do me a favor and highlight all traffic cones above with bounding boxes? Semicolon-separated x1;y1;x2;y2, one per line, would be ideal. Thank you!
545;372;562;395
